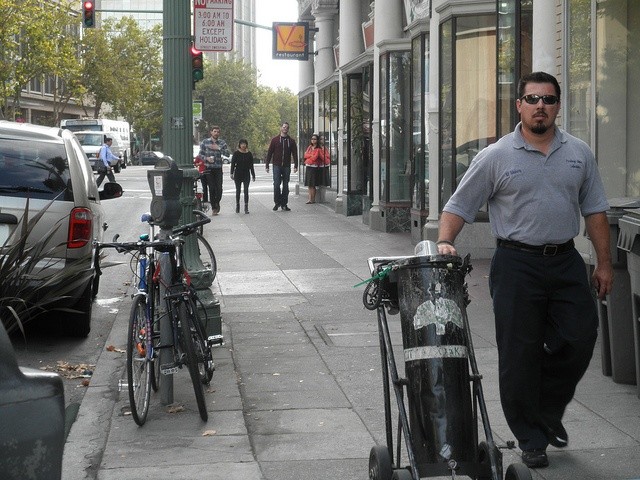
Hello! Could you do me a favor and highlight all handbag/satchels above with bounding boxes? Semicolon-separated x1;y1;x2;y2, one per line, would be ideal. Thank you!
94;146;111;171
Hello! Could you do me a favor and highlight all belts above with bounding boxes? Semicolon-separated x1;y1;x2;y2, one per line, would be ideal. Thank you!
500;241;576;256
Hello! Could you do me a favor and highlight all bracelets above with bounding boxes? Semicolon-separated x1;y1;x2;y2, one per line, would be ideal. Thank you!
437;240;455;246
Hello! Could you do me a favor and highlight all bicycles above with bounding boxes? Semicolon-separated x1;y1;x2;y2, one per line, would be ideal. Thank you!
94;234;185;427
113;210;217;422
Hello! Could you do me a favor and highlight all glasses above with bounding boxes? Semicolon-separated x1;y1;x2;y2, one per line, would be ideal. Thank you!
519;94;557;104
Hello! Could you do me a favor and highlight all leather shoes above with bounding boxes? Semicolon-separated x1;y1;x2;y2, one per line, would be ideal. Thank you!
548;425;568;447
521;450;549;468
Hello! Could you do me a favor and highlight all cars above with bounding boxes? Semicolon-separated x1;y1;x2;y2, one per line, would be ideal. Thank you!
132;151;164;165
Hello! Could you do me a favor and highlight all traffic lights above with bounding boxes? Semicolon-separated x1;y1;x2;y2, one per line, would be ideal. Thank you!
192;35;203;81
82;0;95;27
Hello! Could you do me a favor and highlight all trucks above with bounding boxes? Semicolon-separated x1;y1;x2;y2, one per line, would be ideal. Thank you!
60;119;131;169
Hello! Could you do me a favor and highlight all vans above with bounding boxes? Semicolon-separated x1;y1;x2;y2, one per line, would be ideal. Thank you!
73;131;121;173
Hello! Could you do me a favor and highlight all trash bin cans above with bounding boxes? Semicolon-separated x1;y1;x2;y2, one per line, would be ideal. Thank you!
614;205;640;393
581;198;639;379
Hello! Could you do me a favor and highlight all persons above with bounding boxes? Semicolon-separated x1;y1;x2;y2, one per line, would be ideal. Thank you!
304;135;320;203
265;123;298;211
231;140;255;213
436;72;614;469
195;155;208;202
95;138;121;187
320;136;331;204
199;127;228;215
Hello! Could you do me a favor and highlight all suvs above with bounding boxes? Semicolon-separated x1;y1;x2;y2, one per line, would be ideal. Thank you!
193;145;230;164
0;119;123;338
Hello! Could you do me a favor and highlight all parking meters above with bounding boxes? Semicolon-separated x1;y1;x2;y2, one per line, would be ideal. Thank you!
147;156;183;405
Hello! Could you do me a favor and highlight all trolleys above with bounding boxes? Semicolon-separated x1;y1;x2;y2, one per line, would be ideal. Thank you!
367;240;533;480
